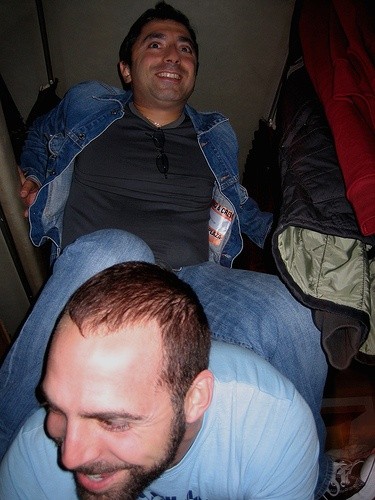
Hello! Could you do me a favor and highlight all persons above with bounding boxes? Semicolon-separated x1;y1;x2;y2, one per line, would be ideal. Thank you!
0;0;375;500
0;261;320;500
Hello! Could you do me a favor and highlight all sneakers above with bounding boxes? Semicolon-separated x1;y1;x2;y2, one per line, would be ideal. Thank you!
318;453;375;500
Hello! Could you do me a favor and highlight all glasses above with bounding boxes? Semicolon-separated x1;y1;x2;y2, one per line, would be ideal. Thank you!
144;127;169;179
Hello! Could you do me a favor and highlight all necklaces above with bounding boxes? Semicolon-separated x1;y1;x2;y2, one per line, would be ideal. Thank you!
145;115;161;127
